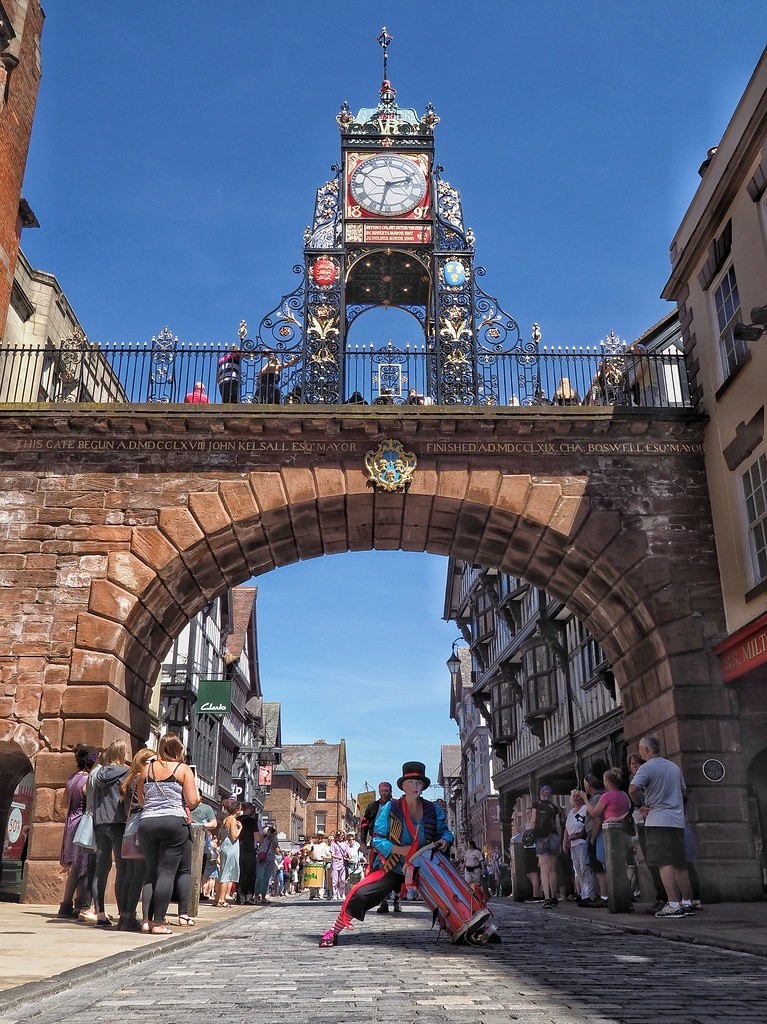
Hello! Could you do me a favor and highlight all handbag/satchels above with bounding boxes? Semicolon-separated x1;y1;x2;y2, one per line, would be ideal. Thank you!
465;865;480;872
72;814;97;850
185;821;195;843
621;814;636;836
258;852;267;861
121;832;144;859
522;829;535;847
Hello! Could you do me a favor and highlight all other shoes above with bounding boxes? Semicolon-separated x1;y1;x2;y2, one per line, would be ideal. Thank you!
394;900;402;912
474;926;501;942
377;904;389;912
318;930;338;947
651;900;666;914
508;894;513;898
524;892;641;909
199;892;271;908
57;901;196;935
309;897;346;900
272;890;303;896
645;900;659;913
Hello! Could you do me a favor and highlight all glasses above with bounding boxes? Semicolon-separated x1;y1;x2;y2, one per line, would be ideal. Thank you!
143;759;155;763
349;839;355;841
342;835;344;836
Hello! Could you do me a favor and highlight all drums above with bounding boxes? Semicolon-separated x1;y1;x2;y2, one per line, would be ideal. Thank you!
401;842;491;945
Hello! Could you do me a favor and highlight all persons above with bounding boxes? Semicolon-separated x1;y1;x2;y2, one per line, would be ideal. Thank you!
629;733;695;918
91;737;131;926
72;747;112;923
56;742;104;923
186;751;703;916
136;733;198;935
319;760;502;946
153;803;196;927
115;746;158;931
177;344;643;409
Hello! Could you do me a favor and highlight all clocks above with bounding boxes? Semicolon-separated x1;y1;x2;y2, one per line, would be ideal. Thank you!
350;154;427;217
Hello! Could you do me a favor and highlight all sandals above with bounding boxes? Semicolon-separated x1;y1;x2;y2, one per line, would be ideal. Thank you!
692;903;704;910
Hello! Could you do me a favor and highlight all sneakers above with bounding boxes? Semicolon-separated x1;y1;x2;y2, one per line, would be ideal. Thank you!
682;904;695;915
655;903;685;917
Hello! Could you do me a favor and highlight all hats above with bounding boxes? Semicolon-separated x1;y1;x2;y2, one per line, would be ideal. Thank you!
397;761;430;792
317;830;326;836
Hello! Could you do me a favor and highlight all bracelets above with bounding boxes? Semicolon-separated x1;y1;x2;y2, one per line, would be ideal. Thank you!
635;802;643;809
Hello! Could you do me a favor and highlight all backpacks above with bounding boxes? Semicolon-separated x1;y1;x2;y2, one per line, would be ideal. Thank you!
532;800;553;839
291;858;298;869
